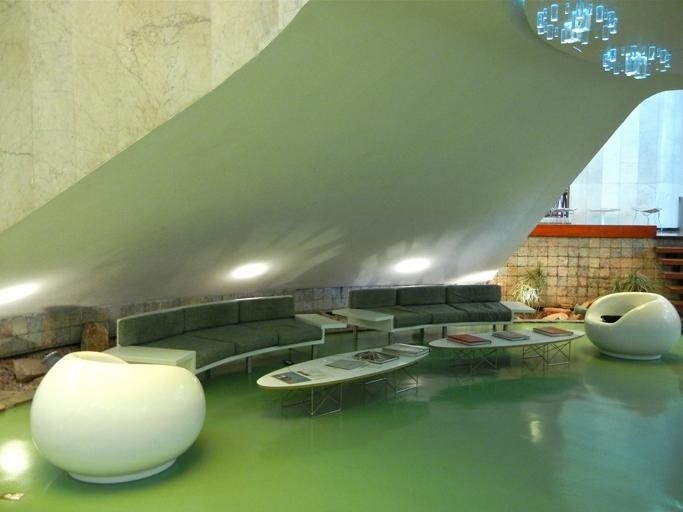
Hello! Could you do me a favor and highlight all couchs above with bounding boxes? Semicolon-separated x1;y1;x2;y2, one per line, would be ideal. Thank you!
102;294;348;384
332;283;539;347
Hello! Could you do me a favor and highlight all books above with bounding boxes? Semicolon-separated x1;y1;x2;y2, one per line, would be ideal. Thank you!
492;329;529;342
446;333;492;347
381;341;431;357
532;326;573;338
599;314;621;324
352;351;399;366
326;360;366;370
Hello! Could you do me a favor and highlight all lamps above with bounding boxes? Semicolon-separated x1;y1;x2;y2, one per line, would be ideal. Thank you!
599;35;673;83
536;1;619;53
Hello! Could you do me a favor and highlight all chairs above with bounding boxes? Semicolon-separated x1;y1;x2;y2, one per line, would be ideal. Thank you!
583;288;682;363
28;347;208;483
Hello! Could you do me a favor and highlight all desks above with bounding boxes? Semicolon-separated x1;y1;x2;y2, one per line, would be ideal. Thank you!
547;205;664;235
